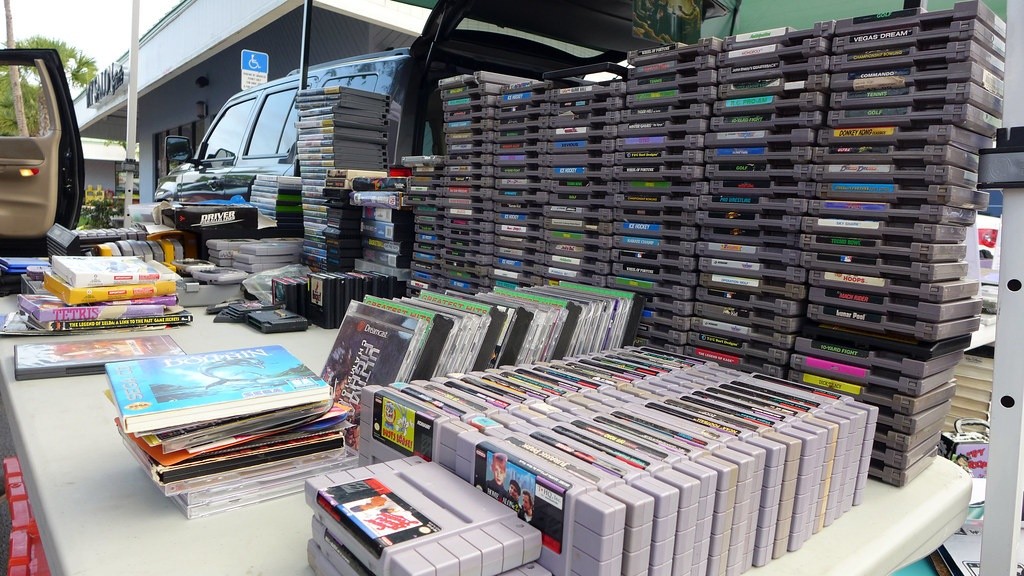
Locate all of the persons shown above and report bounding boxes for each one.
[484,453,533,525]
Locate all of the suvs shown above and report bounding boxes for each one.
[151,1,663,226]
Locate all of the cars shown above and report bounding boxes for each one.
[0,48,86,257]
